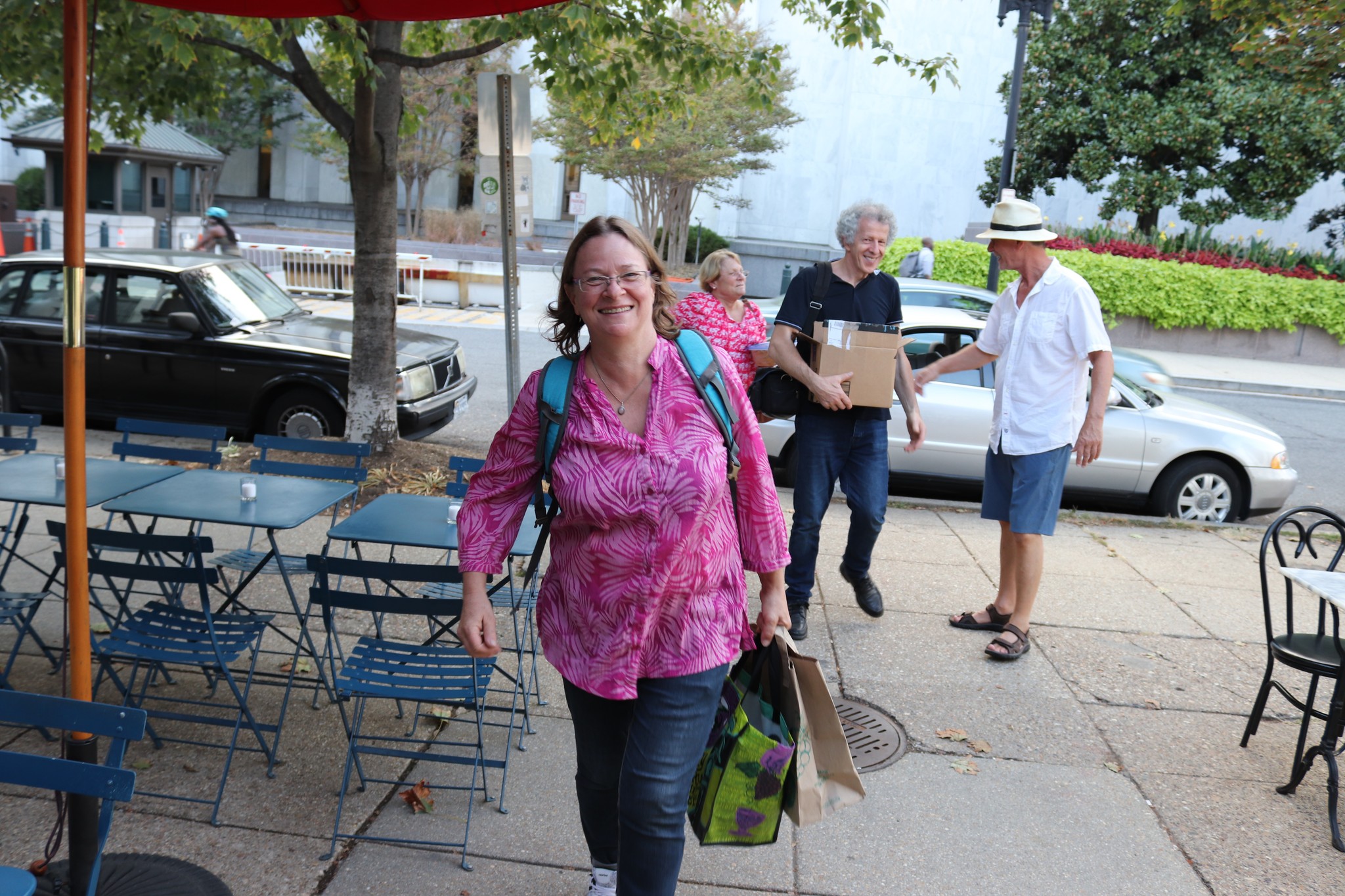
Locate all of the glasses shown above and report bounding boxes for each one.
[720,270,750,279]
[572,271,650,293]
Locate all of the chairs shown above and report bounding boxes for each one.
[929,340,953,358]
[1239,506,1345,798]
[0,411,569,896]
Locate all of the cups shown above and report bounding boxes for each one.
[239,476,257,501]
[447,498,465,524]
[54,456,65,480]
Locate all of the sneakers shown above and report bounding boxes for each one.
[588,866,616,896]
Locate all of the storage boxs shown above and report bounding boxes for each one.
[792,320,916,409]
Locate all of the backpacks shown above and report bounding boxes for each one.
[899,249,924,278]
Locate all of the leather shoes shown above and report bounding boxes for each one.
[839,561,884,618]
[787,605,810,639]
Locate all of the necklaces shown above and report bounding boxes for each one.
[587,343,653,415]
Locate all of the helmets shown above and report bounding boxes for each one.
[203,206,228,219]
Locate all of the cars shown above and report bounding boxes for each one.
[0,249,478,442]
[760,309,1300,525]
[749,275,1172,396]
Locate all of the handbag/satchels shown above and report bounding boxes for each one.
[685,623,866,848]
[748,367,808,420]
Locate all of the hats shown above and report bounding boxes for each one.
[976,198,1058,242]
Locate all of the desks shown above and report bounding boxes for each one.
[0,451,190,742]
[1276,566,1345,854]
[99,466,356,779]
[326,494,561,754]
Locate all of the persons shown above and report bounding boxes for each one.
[910,198,1114,662]
[769,202,924,642]
[189,206,241,273]
[457,213,795,895]
[914,236,934,278]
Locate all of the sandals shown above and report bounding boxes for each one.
[985,623,1031,659]
[948,604,1012,632]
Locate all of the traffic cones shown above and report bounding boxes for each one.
[22,217,36,252]
[197,227,204,252]
[0,225,6,256]
[116,229,125,249]
